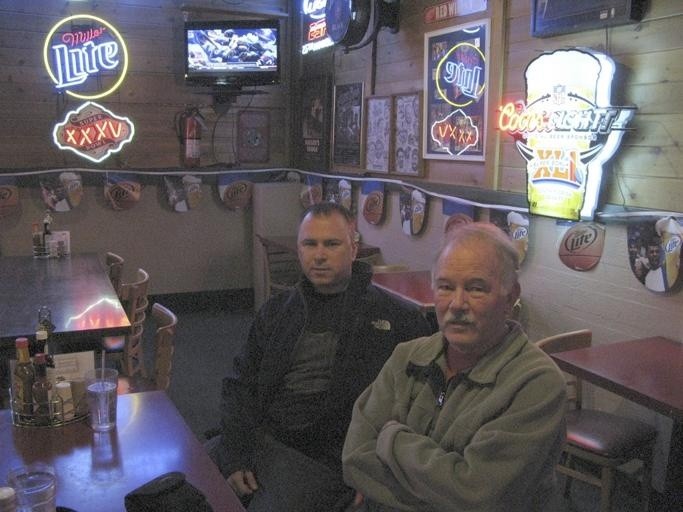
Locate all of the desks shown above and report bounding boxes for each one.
[278,236,380,261]
[549,336,682,512]
[1,252,132,406]
[1,390,244,512]
[372,270,438,336]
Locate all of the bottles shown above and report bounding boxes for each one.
[9,329,86,423]
[0,486,17,511]
[31,219,53,256]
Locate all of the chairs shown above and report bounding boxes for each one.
[71,268,149,377]
[537,329,658,510]
[260,234,303,304]
[150,301,177,396]
[105,252,124,294]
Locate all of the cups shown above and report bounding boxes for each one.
[516,49,608,220]
[64,176,82,206]
[84,365,120,434]
[339,185,353,212]
[182,180,201,209]
[412,197,426,235]
[662,230,681,291]
[7,461,57,512]
[511,221,529,262]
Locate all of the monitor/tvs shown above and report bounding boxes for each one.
[184,19,280,85]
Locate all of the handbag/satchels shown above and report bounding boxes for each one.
[125,470,216,512]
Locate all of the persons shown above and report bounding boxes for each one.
[340,222,567,510]
[626,223,680,291]
[202,203,433,511]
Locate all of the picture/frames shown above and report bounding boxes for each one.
[391,89,428,177]
[235,108,271,165]
[362,93,390,175]
[331,80,365,166]
[293,72,331,173]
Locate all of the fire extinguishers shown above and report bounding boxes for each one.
[175,106,207,169]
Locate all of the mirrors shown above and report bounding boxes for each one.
[422,14,488,164]
